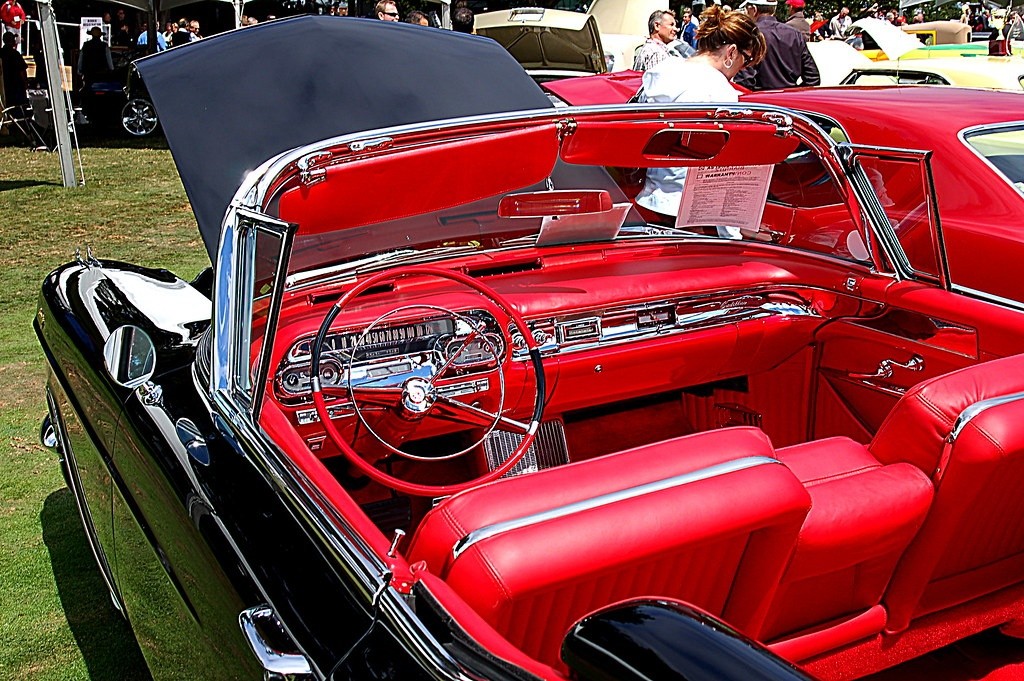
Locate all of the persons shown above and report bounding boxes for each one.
[632,4,768,240]
[0,1,475,143]
[632,0,1024,97]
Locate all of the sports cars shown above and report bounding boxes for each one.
[34,0,1024,681]
